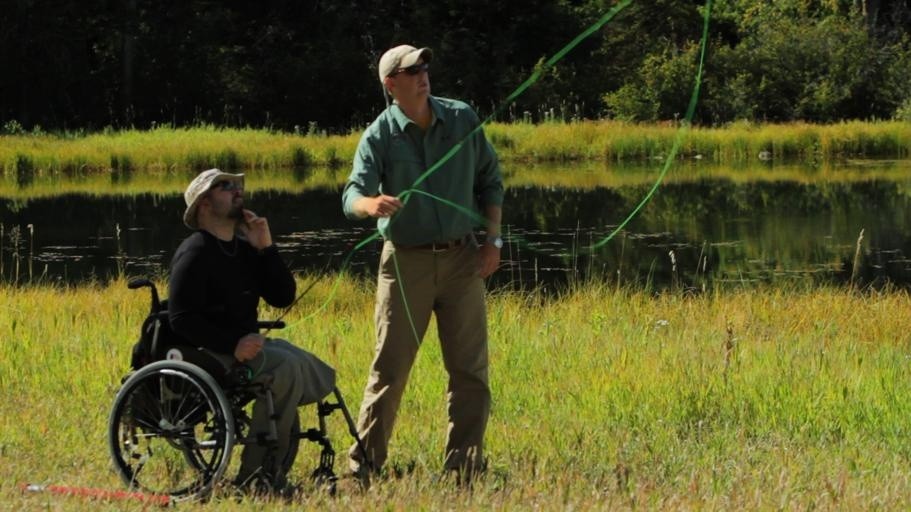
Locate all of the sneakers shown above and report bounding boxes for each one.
[440,467,479,489]
[231,476,301,503]
[344,470,370,494]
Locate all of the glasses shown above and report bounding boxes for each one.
[389,62,428,78]
[220,181,240,191]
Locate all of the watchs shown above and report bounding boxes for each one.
[482,234,504,249]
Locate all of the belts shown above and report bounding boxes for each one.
[418,236,472,252]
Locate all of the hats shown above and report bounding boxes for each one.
[379,45,433,83]
[184,168,245,230]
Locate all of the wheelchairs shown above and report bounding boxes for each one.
[108,276,375,501]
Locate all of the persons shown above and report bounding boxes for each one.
[165,168,337,502]
[340,45,506,490]
